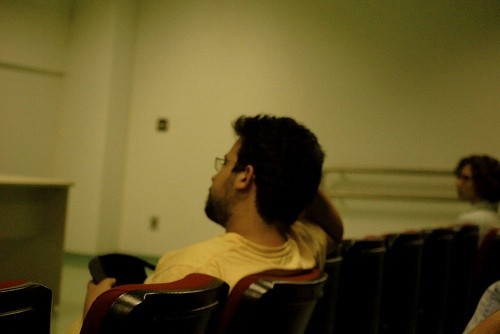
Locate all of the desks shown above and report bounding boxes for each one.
[0,171,74,309]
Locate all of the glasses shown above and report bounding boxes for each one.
[457,174,472,185]
[214,156,237,172]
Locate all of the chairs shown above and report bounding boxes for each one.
[1,217,499,334]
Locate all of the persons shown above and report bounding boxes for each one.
[81,111,331,325]
[451,153,500,248]
[463,279,500,334]
[296,183,346,257]
